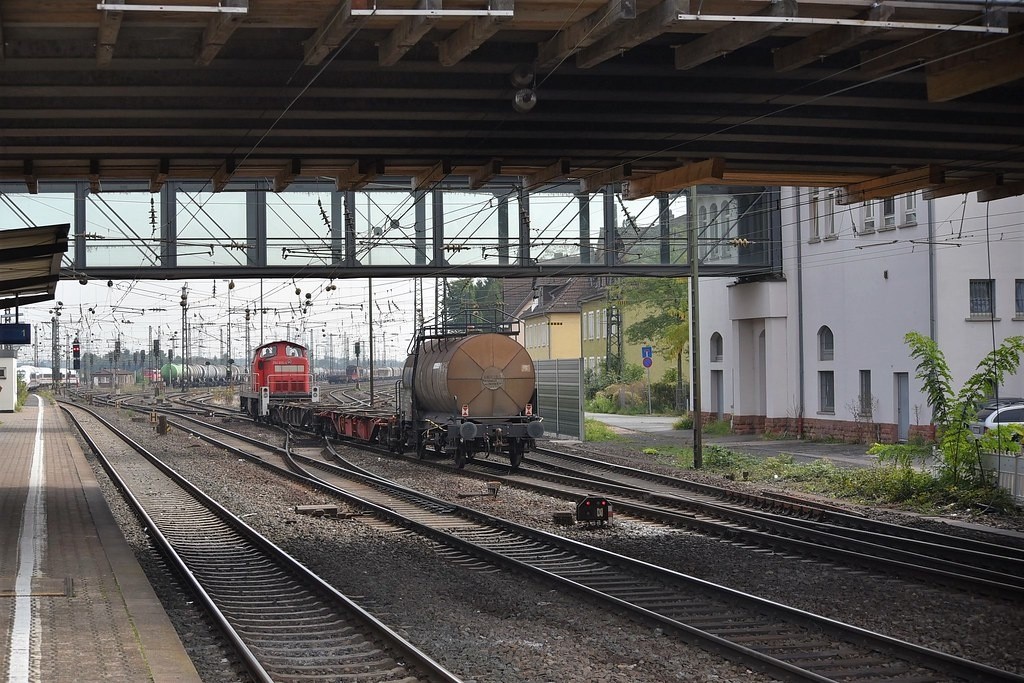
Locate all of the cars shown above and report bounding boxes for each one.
[968,404,1024,454]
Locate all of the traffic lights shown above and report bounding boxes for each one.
[73,345,80,358]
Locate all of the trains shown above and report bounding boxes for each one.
[161,362,402,389]
[237,308,546,468]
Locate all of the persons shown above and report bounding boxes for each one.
[264,349,272,355]
